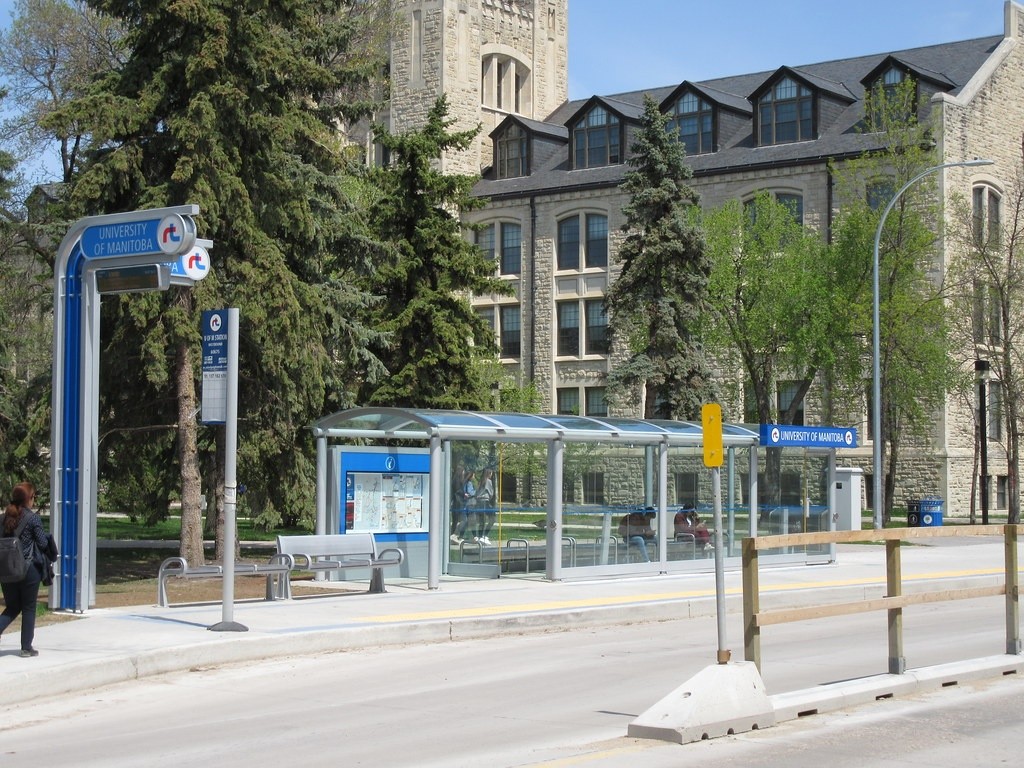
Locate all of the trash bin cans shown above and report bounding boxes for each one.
[904,499,945,527]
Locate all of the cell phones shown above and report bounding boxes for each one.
[703,520,706,525]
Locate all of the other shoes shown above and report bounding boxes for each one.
[704,544,715,551]
[450,534,461,544]
[474,536,478,542]
[21,647,38,657]
[479,536,492,545]
[458,538,469,545]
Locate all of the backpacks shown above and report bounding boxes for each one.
[617,513,641,536]
[0,511,35,584]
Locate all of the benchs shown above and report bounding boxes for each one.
[276,532,404,597]
[157,552,294,611]
[458,530,731,578]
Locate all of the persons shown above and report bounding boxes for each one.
[673,500,715,553]
[0,482,58,658]
[476,467,497,545]
[450,465,469,541]
[458,469,479,541]
[618,507,659,563]
